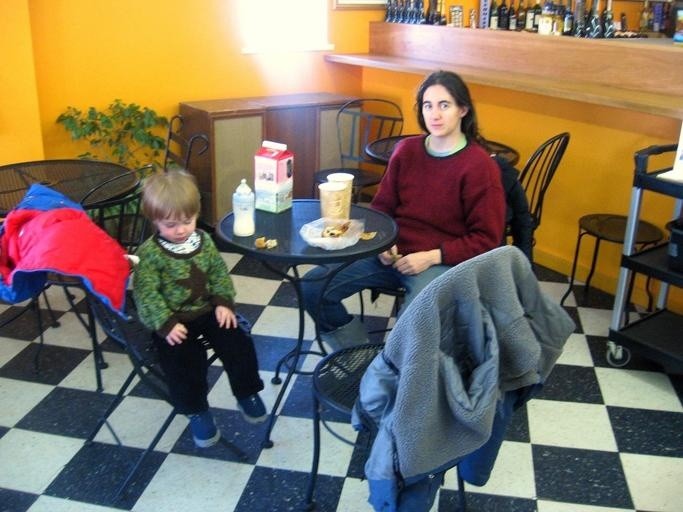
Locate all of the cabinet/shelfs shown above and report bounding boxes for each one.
[179,92,361,232]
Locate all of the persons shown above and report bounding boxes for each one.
[294,71,507,392]
[132,168,271,449]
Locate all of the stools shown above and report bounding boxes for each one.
[560,213,664,326]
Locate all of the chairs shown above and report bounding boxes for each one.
[79,278,251,504]
[301,245,574,512]
[376,132,571,294]
[310,98,404,204]
[2,115,211,394]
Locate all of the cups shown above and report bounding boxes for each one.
[319,172,355,228]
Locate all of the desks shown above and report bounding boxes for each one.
[364,135,520,171]
[218,198,400,450]
[1,158,141,368]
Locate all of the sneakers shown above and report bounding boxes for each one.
[189,408,221,450]
[235,391,268,424]
[315,349,378,400]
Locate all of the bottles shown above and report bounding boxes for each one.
[485,0,682,43]
[230,179,257,238]
[382,0,481,33]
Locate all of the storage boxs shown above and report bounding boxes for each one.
[666,220,682,274]
